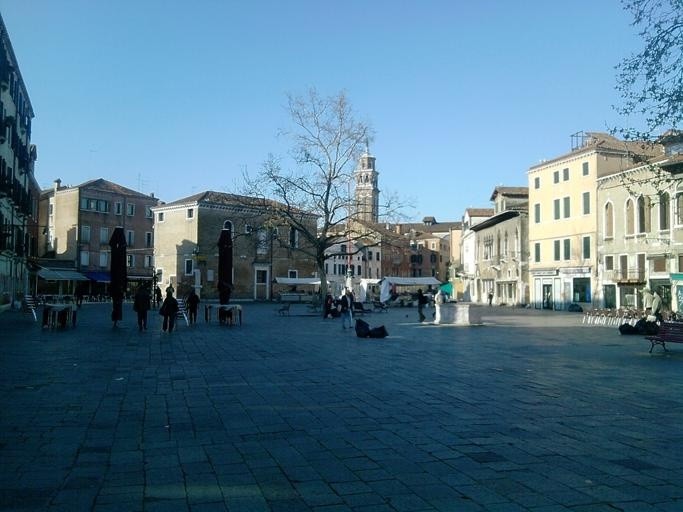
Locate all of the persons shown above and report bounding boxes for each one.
[165,283,174,297]
[433,288,442,322]
[323,290,356,322]
[486,288,494,305]
[156,287,161,308]
[132,283,153,331]
[158,292,178,332]
[417,288,426,322]
[340,289,353,329]
[185,287,200,325]
[651,290,663,316]
[73,281,84,309]
[638,287,653,314]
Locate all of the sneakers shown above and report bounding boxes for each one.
[139,327,148,330]
[418,316,425,322]
[343,326,353,329]
[190,321,196,325]
[161,327,172,331]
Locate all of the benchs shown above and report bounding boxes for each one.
[645,322,683,353]
[336,300,387,317]
